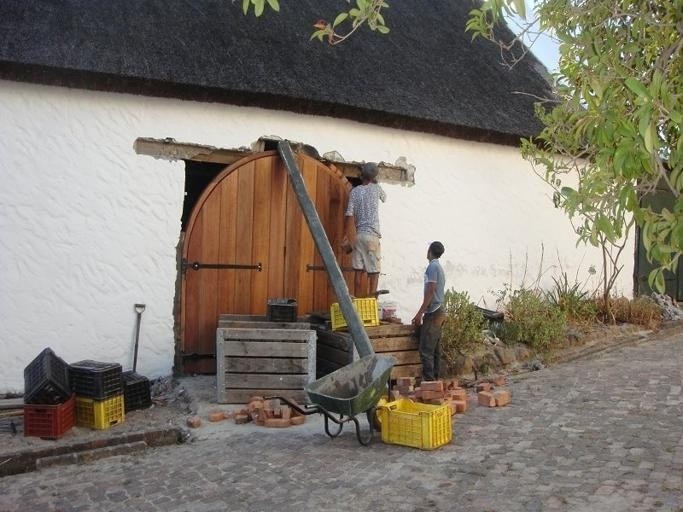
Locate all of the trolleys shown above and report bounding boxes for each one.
[262,353,396,446]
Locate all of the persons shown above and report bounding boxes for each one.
[412,241,445,381]
[342,162,387,299]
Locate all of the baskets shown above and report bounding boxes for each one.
[331,298,380,332]
[376,398,453,451]
[267,298,297,322]
[24,346,153,439]
